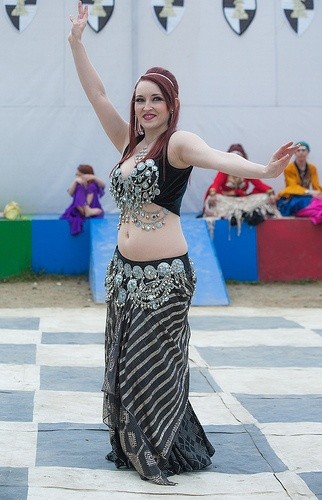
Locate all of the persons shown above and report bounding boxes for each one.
[68,164,106,217]
[69,0,301,486]
[277,141,322,216]
[203,143,276,215]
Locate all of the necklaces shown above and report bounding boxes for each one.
[134,135,161,165]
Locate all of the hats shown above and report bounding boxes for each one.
[293,140,310,151]
